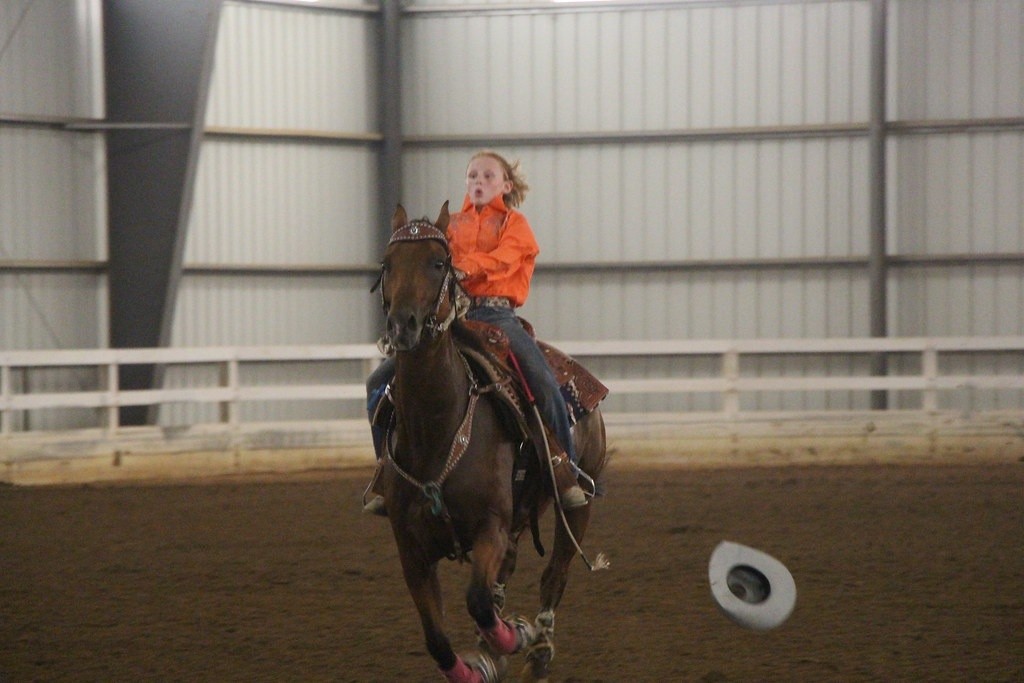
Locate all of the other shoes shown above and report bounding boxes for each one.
[561,482,589,511]
[362,494,386,518]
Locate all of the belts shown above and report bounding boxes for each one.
[467,295,514,308]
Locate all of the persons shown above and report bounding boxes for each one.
[351,145,591,517]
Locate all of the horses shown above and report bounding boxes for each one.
[377,198,619,683]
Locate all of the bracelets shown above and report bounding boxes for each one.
[459,269,467,280]
